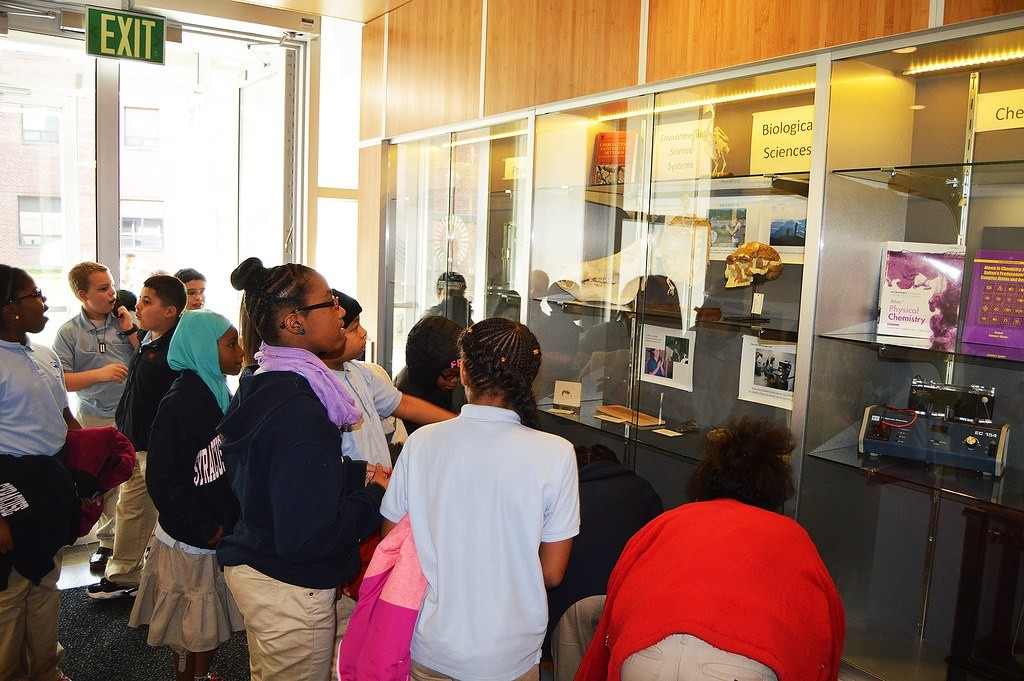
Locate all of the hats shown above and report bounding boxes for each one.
[332,289,362,330]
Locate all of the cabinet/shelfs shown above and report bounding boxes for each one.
[389,156,1024,472]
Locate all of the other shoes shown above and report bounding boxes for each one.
[195,670,226,681]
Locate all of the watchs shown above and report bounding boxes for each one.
[122,323,138,336]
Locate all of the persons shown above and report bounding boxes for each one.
[52,261,155,569]
[521,269,743,430]
[86,275,188,599]
[726,218,741,248]
[429,272,474,328]
[548,444,667,681]
[175,268,207,310]
[665,339,679,361]
[386,316,468,468]
[0,263,106,681]
[647,348,665,377]
[756,353,774,378]
[320,288,458,681]
[379,318,580,681]
[214,257,391,681]
[128,308,245,643]
[573,419,846,681]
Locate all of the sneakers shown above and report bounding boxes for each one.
[89,547,113,567]
[86,578,139,599]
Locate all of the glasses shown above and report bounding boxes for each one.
[280,298,338,328]
[2,288,42,312]
[441,371,460,380]
